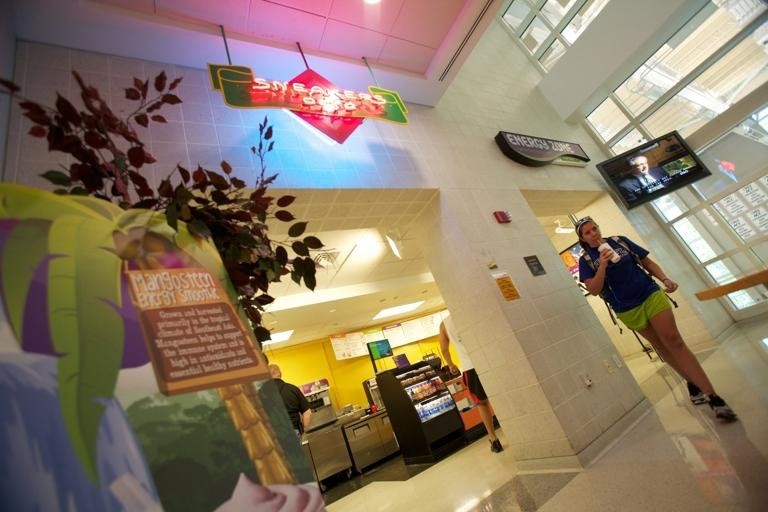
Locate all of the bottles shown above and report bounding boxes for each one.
[404,377,456,424]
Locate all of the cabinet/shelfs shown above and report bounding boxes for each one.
[375,360,467,465]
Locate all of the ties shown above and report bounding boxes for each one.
[642,175,657,187]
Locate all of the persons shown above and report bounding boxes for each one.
[258,363,313,448]
[618,153,673,204]
[439,313,505,453]
[213,471,326,512]
[675,160,690,172]
[574,213,740,424]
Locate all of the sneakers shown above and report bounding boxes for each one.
[687,383,734,418]
[489,439,502,452]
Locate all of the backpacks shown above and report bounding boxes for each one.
[582,235,641,302]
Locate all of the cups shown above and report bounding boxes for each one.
[365,406,379,415]
[597,241,621,264]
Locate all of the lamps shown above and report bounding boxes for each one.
[385,225,408,260]
[554,219,575,234]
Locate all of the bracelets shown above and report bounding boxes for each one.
[662,278,669,283]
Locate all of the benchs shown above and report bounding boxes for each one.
[695,267,768,301]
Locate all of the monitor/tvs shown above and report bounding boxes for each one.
[368,338,393,361]
[596,129,712,210]
